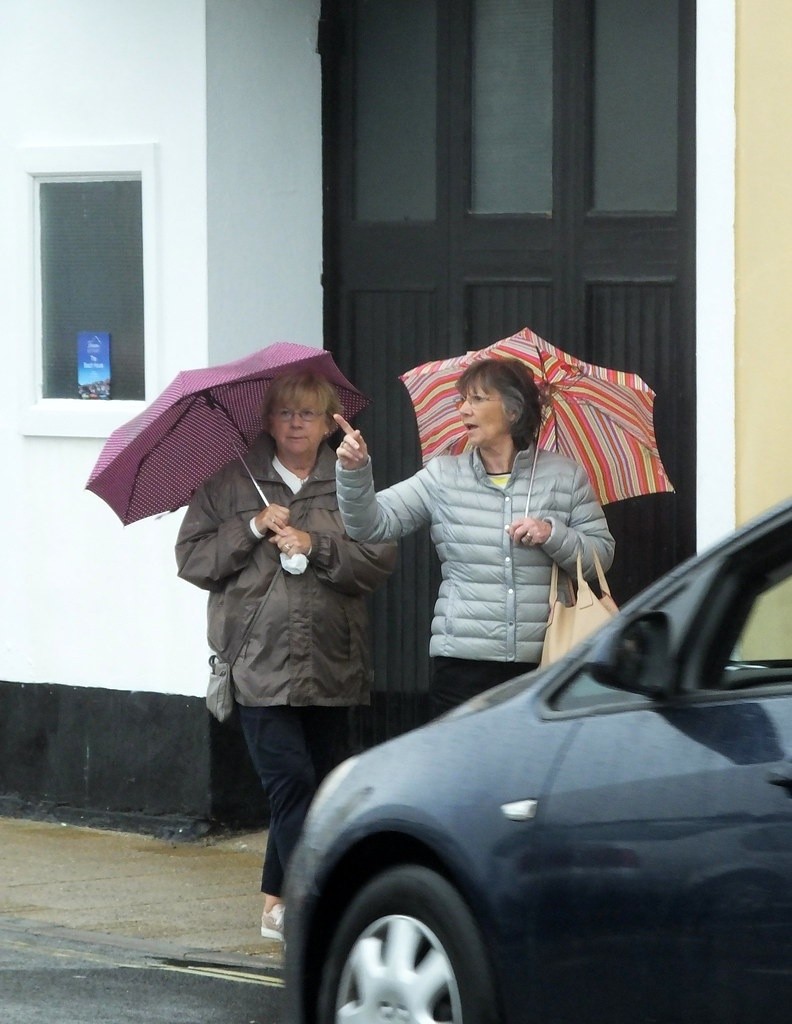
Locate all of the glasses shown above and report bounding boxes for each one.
[269,407,324,422]
[453,395,501,410]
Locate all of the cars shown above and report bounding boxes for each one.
[284,500,792,1023]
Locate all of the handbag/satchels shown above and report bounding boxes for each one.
[540,542,619,668]
[206,655,233,723]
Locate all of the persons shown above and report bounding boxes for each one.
[177,371,395,937]
[332,360,615,713]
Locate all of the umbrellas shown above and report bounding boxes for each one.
[399,328,675,516]
[84,341,373,526]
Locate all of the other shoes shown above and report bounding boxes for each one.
[261,904,285,941]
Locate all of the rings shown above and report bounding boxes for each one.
[285,545,290,549]
[527,533,532,537]
